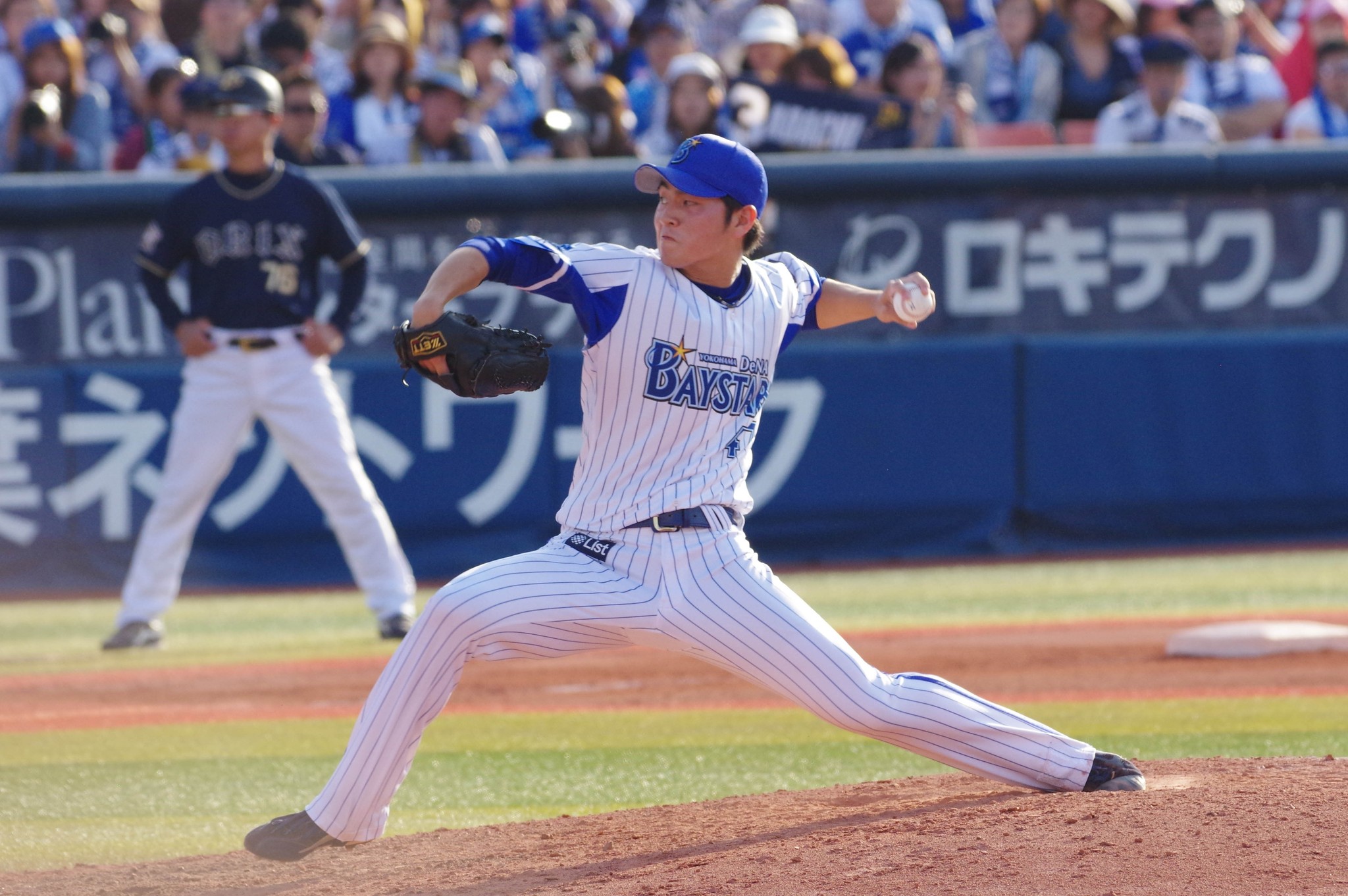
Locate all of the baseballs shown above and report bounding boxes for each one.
[892,282,934,324]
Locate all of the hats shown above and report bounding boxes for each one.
[20,21,69,47]
[1137,37,1189,63]
[739,6,796,48]
[358,14,408,49]
[460,13,502,56]
[203,65,285,116]
[418,56,475,98]
[663,54,716,86]
[634,134,768,220]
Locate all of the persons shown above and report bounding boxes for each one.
[243,136,1147,861]
[102,66,418,649]
[0,0,1348,174]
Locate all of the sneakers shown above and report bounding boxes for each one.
[244,811,372,861]
[381,612,413,638]
[1085,752,1142,794]
[101,623,159,647]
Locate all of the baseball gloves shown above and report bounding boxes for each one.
[394,311,549,400]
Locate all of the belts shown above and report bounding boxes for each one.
[621,506,736,532]
[204,334,301,351]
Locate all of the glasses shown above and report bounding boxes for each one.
[287,100,326,116]
